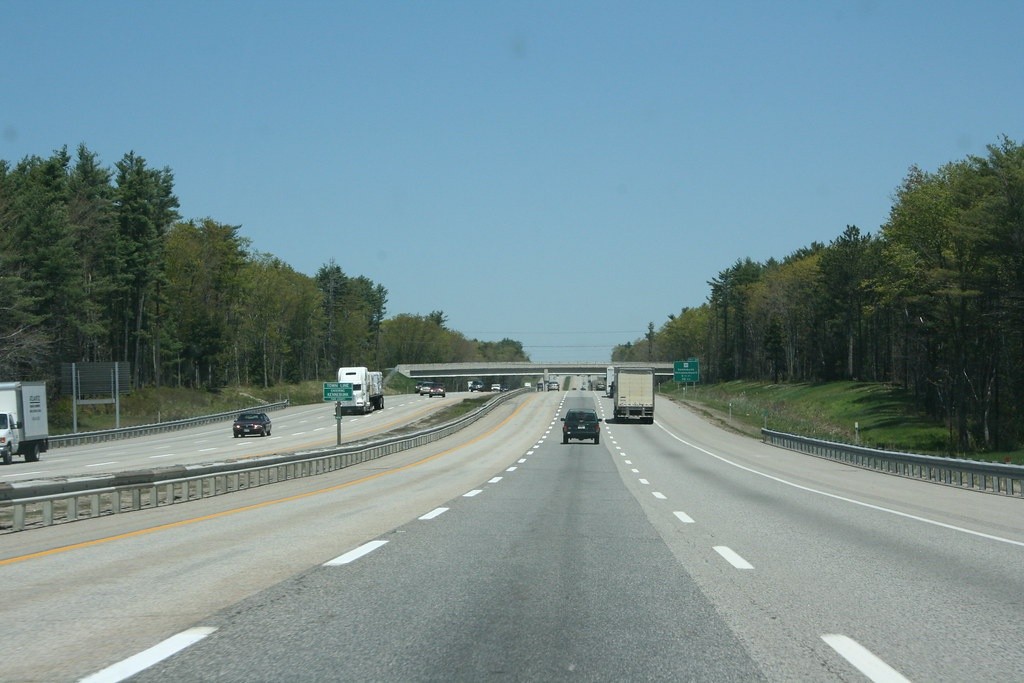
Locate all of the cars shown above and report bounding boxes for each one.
[524,382,532,390]
[570,384,606,391]
[233,412,272,437]
[414,381,424,394]
[490,383,501,393]
[500,384,513,392]
[469,381,485,393]
[537,382,543,388]
[547,382,560,391]
[609,381,613,398]
[560,409,603,445]
[418,380,434,397]
[428,384,446,397]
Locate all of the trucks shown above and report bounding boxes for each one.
[609,365,655,425]
[0,381,50,464]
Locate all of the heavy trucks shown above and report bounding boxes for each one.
[333,367,385,415]
